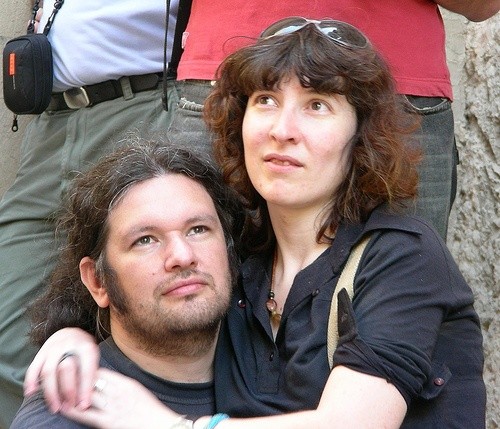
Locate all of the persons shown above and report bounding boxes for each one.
[160,1,499,250]
[10,141,255,429]
[0,1,193,399]
[24,17,487,429]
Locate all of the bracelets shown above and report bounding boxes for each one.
[202,413,230,429]
[177,412,200,428]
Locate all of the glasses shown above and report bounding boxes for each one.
[258,15,369,49]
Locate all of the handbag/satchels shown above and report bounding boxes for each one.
[3,33,53,132]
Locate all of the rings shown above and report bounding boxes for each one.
[54,352,77,364]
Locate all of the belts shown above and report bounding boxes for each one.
[45,71,176,110]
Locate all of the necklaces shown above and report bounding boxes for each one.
[266,247,282,325]
